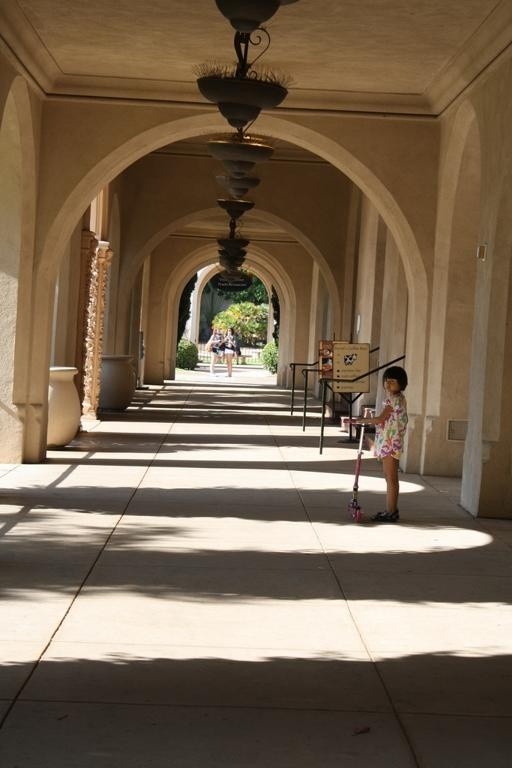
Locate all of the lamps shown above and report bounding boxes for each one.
[186,1,290,277]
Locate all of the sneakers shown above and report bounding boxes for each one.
[371,510,399,522]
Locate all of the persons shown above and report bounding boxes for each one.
[348,365,408,524]
[208,327,224,376]
[222,328,237,378]
